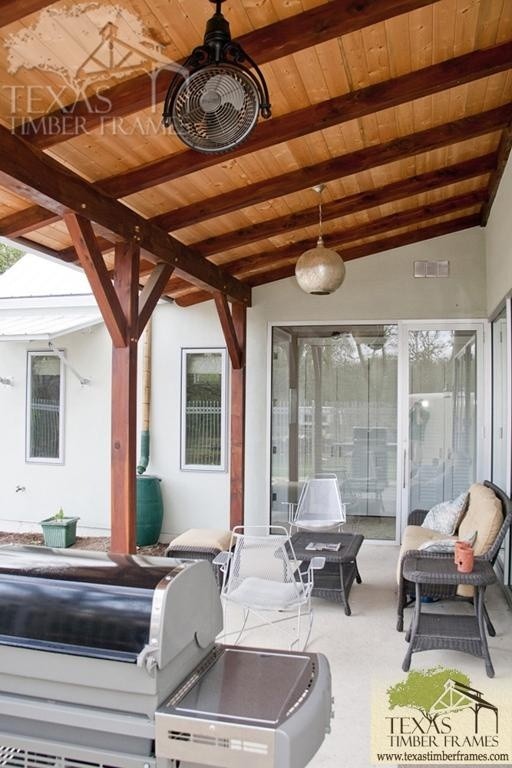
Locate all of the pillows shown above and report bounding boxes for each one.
[418,530,478,553]
[420,491,469,534]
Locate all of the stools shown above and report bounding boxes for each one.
[163,526,239,589]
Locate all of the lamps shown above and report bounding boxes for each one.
[293,185,346,294]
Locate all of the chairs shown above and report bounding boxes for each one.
[278,427,398,522]
[211,523,329,656]
[281,469,349,537]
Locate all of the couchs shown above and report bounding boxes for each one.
[412,451,473,513]
[395,478,512,634]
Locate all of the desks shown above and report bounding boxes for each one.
[402,553,499,678]
[276,527,364,618]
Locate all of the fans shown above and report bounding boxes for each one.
[161,0,273,153]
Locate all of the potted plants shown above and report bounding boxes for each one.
[39,508,80,548]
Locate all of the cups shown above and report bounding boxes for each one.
[457,548,473,575]
[456,541,470,565]
